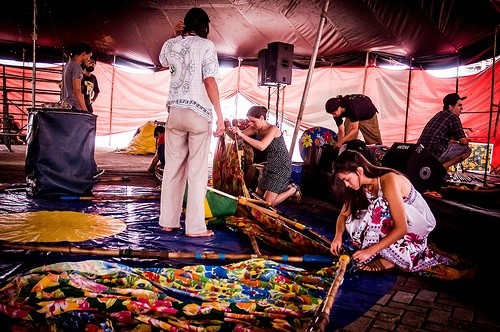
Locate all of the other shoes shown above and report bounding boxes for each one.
[93,169,105,179]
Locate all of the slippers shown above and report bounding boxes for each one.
[364,260,396,272]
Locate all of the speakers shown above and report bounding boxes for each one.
[381,142,448,193]
[256,41,294,87]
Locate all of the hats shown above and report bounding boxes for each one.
[443,93,467,105]
[325,95,343,114]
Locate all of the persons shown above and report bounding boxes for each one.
[9,116,20,128]
[62,42,105,180]
[418,94,472,169]
[148,126,165,171]
[325,92,383,149]
[330,151,451,272]
[224,105,303,207]
[159,7,224,237]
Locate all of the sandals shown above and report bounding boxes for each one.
[290,183,302,205]
